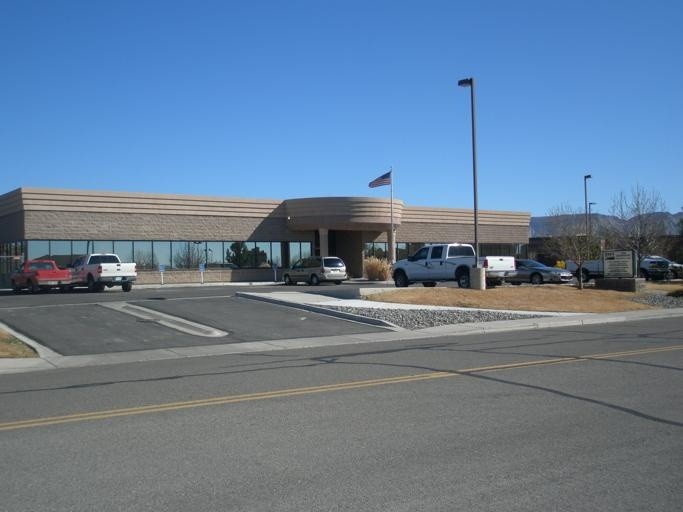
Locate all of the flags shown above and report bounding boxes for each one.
[368,171,392,187]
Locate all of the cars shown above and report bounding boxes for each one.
[647,258,682,280]
[206,263,239,269]
[281,256,347,286]
[493,258,573,286]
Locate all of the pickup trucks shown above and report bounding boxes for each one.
[69,252,137,293]
[390,243,517,288]
[10,260,71,294]
[564,253,668,284]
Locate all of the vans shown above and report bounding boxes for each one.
[534,254,579,277]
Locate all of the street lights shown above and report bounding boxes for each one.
[193,240,201,269]
[588,202,596,258]
[457,77,479,267]
[583,174,592,258]
